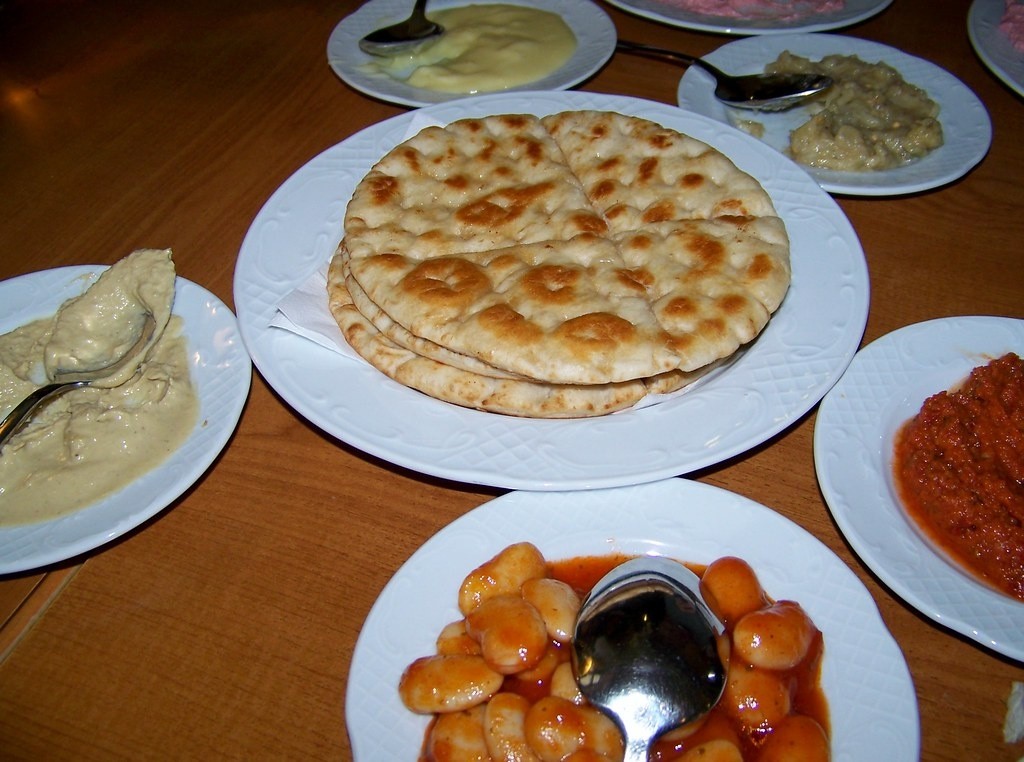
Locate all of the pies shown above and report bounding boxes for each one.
[326,107,795,420]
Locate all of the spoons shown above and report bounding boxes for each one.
[613,39,834,111]
[358,0,446,58]
[0,315,159,452]
[568,556,733,762]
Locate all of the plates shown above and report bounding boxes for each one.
[233,90,868,492]
[342,475,921,762]
[0,264,254,574]
[675,33,993,196]
[605,0,894,37]
[967,0,1024,100]
[326,0,618,108]
[813,316,1024,665]
